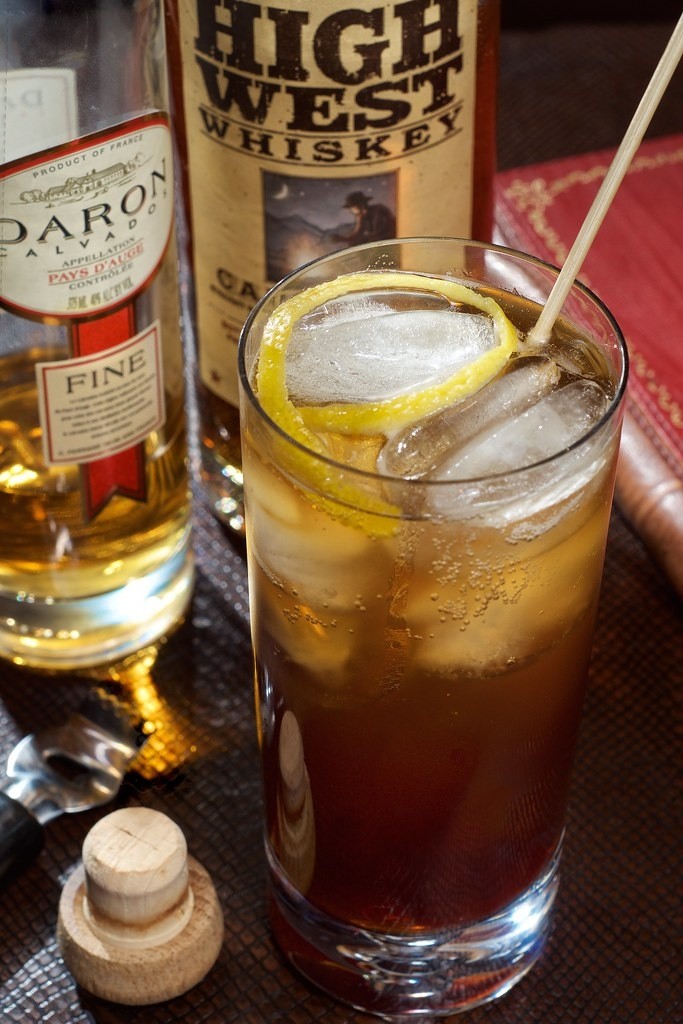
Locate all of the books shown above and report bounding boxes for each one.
[491,132,682,576]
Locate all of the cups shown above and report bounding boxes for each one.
[235,239,629,1024]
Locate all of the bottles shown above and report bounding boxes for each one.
[167,0,500,534]
[0,0,188,670]
